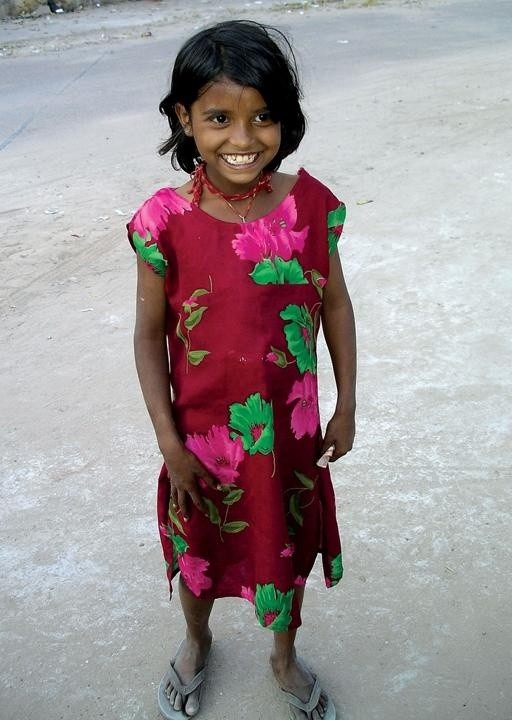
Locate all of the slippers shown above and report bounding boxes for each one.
[158,639,208,720]
[280,655,336,720]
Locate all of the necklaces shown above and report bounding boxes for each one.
[185,160,276,224]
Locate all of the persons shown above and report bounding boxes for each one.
[123,19,360,720]
